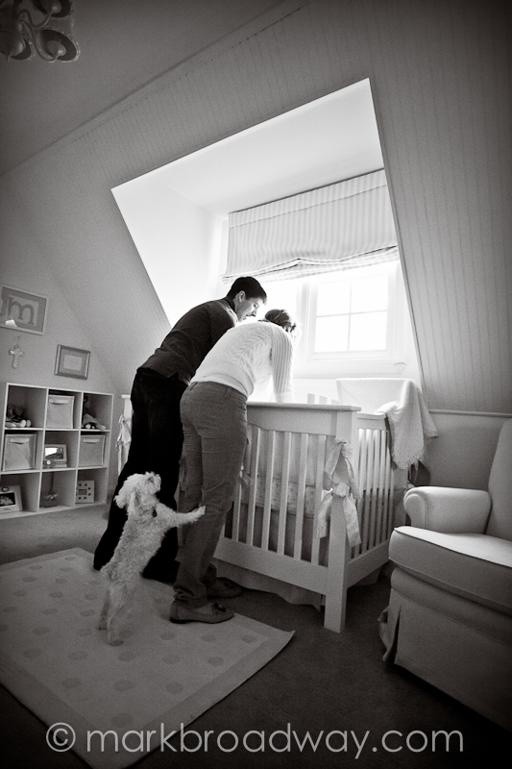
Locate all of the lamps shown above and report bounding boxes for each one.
[0,0,80,63]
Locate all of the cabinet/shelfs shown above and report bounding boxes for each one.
[0,383,114,523]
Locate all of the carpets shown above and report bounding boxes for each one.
[1,548,296,769]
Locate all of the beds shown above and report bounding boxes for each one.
[117,393,426,634]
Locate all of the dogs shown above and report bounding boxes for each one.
[96,471,207,647]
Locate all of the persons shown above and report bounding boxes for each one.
[170,310,297,624]
[94,277,266,584]
[9,302,33,326]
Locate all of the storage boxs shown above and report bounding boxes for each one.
[46,395,75,430]
[75,480,96,503]
[80,435,105,467]
[2,433,37,471]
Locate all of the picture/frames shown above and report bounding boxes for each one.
[0,284,50,336]
[54,344,91,381]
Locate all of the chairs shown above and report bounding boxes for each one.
[376,418,511,734]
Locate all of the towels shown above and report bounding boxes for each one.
[374,380,438,470]
